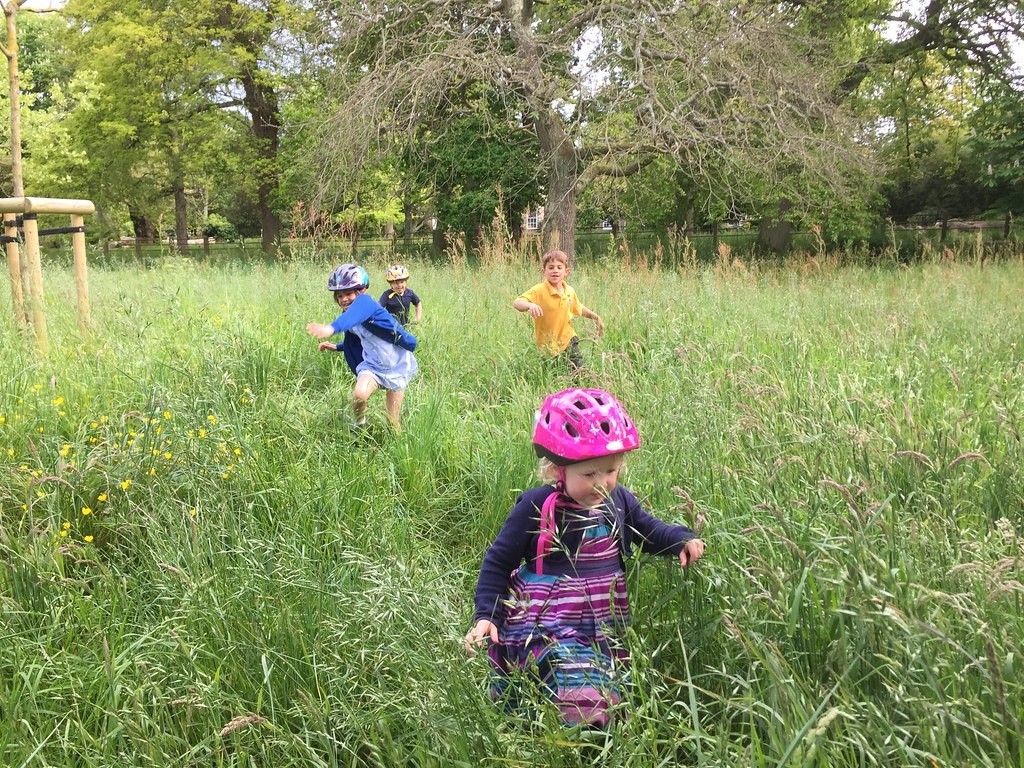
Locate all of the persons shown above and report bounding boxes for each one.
[305,263,418,447]
[377,265,422,331]
[463,386,705,768]
[513,251,605,388]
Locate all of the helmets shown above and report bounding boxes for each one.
[328,264,370,292]
[532,389,640,467]
[386,264,409,282]
